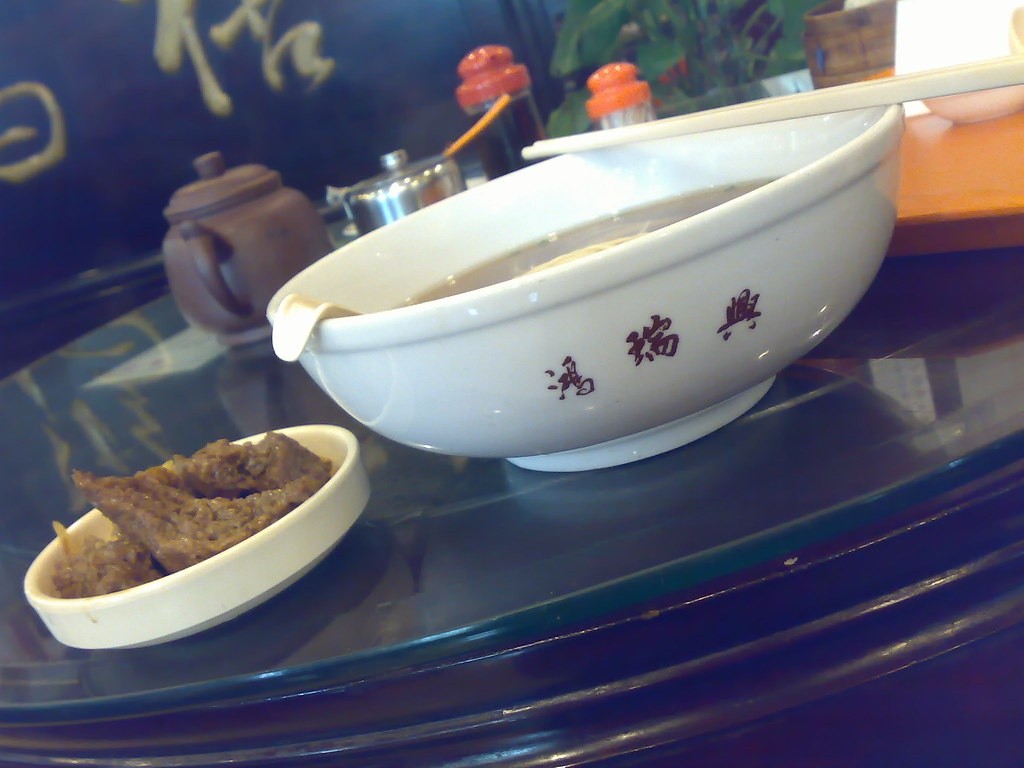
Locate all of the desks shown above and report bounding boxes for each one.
[0,194,1024,768]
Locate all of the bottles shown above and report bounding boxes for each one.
[455,45,550,179]
[585,61,657,130]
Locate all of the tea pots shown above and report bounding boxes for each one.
[162,150,336,347]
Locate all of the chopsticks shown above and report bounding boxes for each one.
[521,50,1023,160]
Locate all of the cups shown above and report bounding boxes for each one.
[340,148,468,236]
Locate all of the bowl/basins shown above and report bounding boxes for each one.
[23,424,372,650]
[264,102,905,473]
